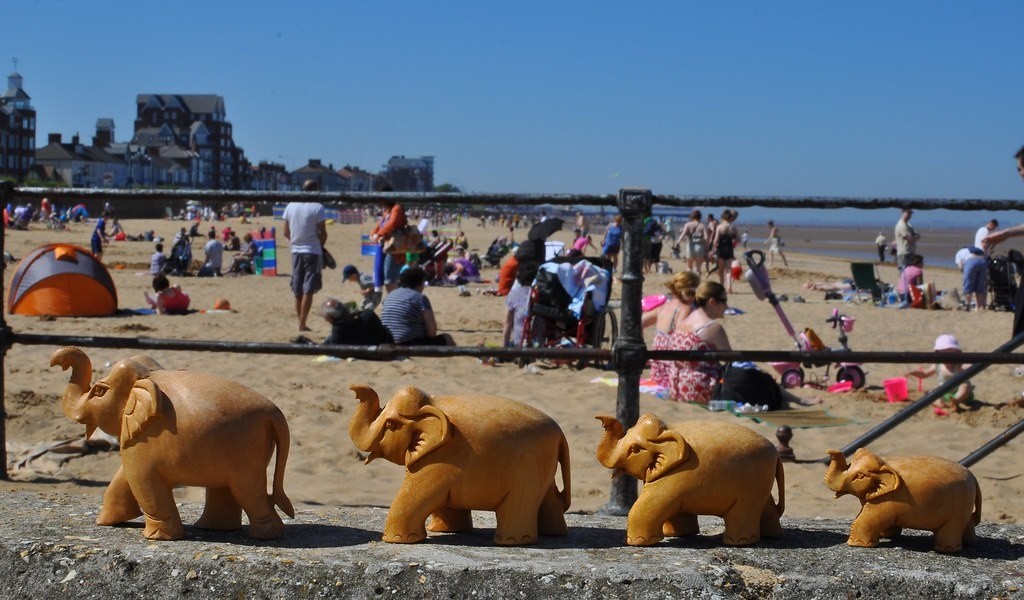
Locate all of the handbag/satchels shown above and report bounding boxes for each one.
[936,287,964,312]
[382,203,425,255]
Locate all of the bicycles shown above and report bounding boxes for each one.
[743,249,869,392]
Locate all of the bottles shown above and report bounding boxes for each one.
[708,400,743,412]
[657,388,671,400]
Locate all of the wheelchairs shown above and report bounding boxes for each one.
[518,255,619,368]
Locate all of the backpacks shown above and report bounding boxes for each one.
[711,362,790,411]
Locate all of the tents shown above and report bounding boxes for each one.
[7,243,118,316]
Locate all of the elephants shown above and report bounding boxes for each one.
[350,384,571,546]
[49,346,294,539]
[826,449,983,550]
[597,414,785,547]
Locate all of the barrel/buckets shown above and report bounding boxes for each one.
[883,378,908,402]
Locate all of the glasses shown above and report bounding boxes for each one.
[719,298,728,305]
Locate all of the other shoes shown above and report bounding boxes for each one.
[321,247,336,270]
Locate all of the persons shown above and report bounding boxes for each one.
[0,146,1024,412]
[151,275,190,315]
[282,179,327,333]
[902,334,975,413]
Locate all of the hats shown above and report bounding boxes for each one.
[902,206,914,212]
[341,265,357,283]
[934,334,961,351]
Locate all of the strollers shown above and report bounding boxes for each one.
[987,255,1019,312]
